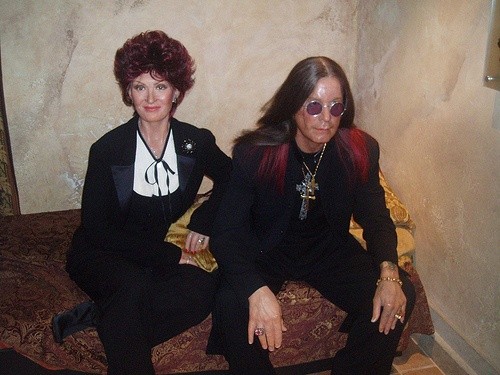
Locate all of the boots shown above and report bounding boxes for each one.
[51,303,96,343]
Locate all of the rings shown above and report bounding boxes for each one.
[198,238,204,243]
[254,327,265,336]
[395,312,403,320]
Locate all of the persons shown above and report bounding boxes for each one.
[50,29,233,375]
[208,56,418,375]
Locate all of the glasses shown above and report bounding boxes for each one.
[302,100,346,117]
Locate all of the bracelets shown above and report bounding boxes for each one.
[376,276,403,287]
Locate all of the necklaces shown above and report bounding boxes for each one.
[290,142,327,221]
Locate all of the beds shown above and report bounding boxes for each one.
[0,194,436,375]
